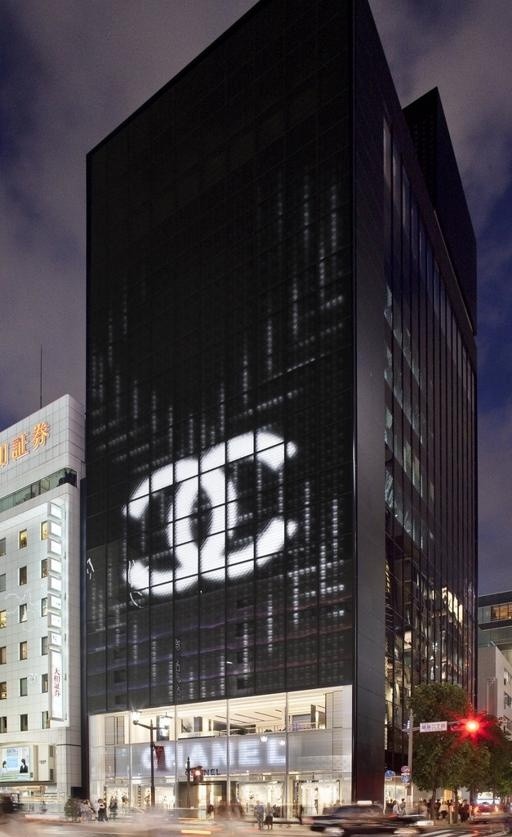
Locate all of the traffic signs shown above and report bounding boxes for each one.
[418,722,448,733]
[401,773,411,784]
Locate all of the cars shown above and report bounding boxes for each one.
[309,802,434,836]
[0,792,21,818]
[468,809,512,825]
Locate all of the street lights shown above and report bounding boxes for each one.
[184,756,204,809]
[132,719,157,811]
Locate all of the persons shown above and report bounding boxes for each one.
[254,799,265,830]
[79,798,119,824]
[385,797,480,824]
[265,802,274,830]
[206,803,214,818]
[40,800,47,814]
[1,759,8,772]
[298,803,304,824]
[20,758,29,773]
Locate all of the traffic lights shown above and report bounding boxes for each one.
[451,719,481,733]
